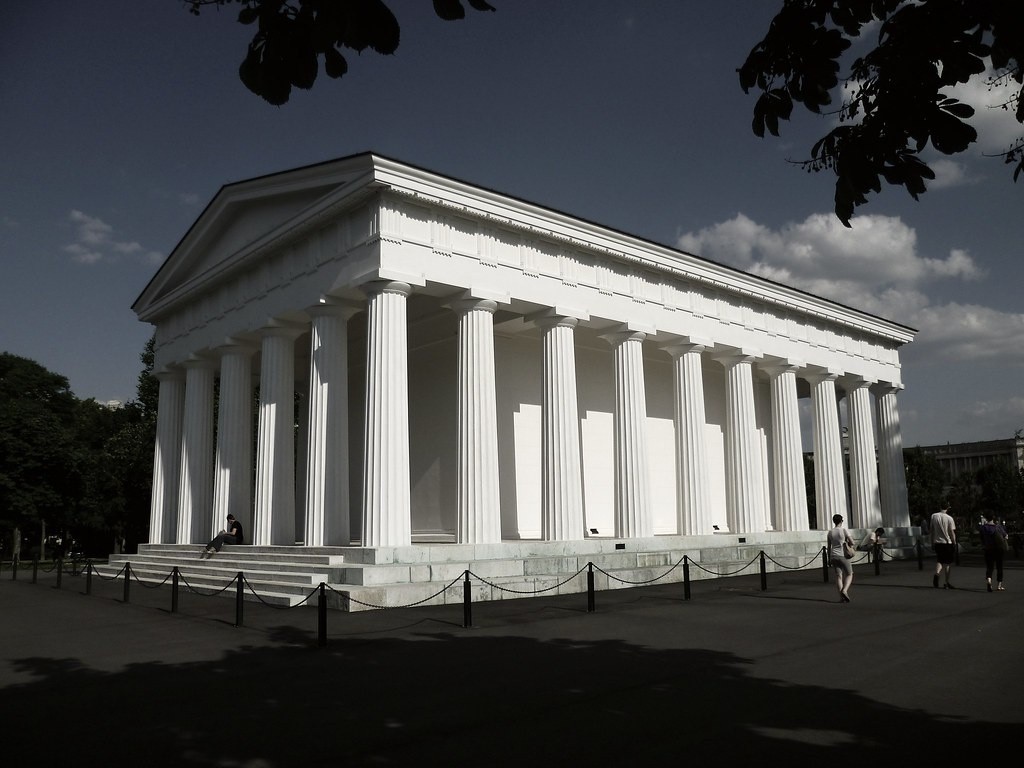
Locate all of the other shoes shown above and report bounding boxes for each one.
[200,547,216,553]
[944,582,956,589]
[933,573,939,587]
[840,591,850,603]
[987,583,992,592]
[997,586,1005,591]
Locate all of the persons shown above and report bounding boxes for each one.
[993,518,1019,533]
[858,527,887,562]
[200,514,243,554]
[827,515,854,603]
[920,516,929,536]
[930,501,959,590]
[980,510,1006,592]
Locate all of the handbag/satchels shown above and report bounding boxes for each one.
[843,542,856,559]
[995,530,1009,551]
